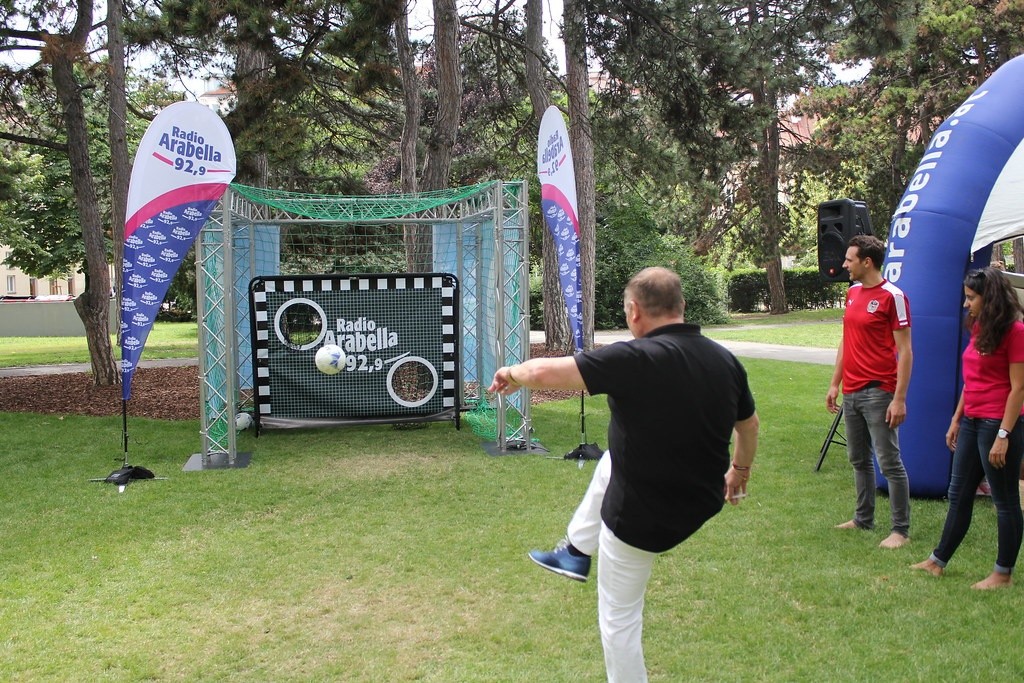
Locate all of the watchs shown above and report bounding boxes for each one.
[998,428,1011,439]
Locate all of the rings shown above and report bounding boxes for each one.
[505,386,508,390]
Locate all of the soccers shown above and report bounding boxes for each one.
[315,345,346,375]
[235,413,253,431]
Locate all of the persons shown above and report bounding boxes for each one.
[826,234,914,549]
[990,261,1005,271]
[909,266,1024,589]
[488,264,759,683]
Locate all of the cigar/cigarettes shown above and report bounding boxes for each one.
[725,494,746,499]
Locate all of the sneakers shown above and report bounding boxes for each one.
[528,536,591,583]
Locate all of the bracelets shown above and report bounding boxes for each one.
[732,460,751,480]
[506,364,522,388]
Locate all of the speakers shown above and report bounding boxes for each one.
[818,198,873,283]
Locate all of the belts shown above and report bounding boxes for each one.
[861,380,881,390]
